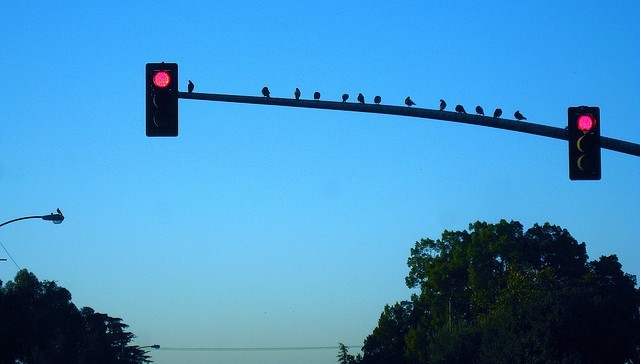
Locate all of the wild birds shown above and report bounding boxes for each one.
[455,104,467,113]
[494,108,503,117]
[475,105,484,115]
[342,93,349,102]
[357,92,364,102]
[261,86,271,96]
[404,95,417,107]
[514,109,527,121]
[374,95,381,105]
[439,98,447,110]
[187,79,195,92]
[293,87,301,99]
[313,91,321,99]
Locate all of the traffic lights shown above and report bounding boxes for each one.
[145,62,178,137]
[567,105,600,179]
